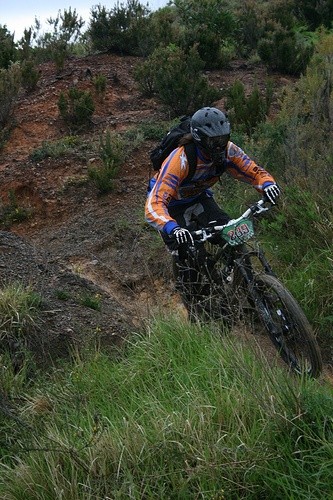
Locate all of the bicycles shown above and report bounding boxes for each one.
[171,198,323,381]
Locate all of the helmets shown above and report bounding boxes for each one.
[191,106,230,160]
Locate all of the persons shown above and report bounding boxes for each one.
[143,107,282,324]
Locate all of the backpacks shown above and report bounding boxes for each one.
[150,115,228,188]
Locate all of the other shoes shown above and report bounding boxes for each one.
[246,291,280,309]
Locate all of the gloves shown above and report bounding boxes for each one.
[263,184,281,205]
[170,226,194,247]
